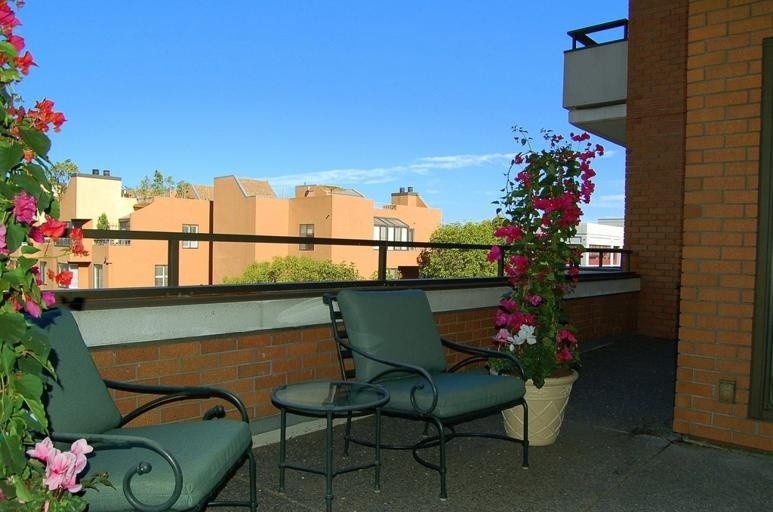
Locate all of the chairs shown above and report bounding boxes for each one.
[319,289,529,506]
[12,304,261,512]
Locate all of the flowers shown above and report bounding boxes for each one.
[16,290,57,322]
[482,121,603,388]
[21,430,93,508]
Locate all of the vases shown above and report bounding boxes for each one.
[491,370,578,448]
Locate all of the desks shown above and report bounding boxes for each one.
[268,380,393,509]
